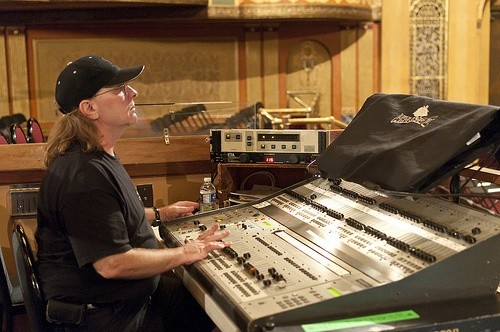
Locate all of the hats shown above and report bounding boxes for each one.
[55,56,145,115]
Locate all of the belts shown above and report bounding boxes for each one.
[84,302,125,309]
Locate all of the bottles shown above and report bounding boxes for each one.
[198,177,216,213]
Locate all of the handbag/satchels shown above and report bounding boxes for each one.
[316,93,500,194]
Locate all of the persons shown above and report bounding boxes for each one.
[34,55,231,332]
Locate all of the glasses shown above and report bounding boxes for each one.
[87,82,127,99]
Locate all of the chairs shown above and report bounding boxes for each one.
[0,118,44,144]
[12,223,47,331]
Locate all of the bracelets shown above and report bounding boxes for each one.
[153,207,160,227]
